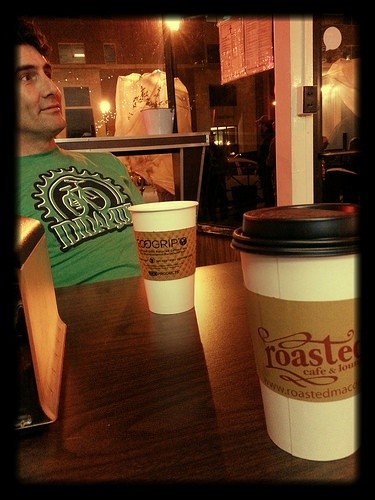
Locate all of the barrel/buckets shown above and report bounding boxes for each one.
[141,107,175,135]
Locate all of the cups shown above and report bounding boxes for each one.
[230,203,360,460]
[127,201,200,314]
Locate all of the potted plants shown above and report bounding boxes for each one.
[128,86,175,135]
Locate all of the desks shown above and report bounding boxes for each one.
[18,261,357,481]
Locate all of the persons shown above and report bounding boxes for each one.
[16,20,145,288]
